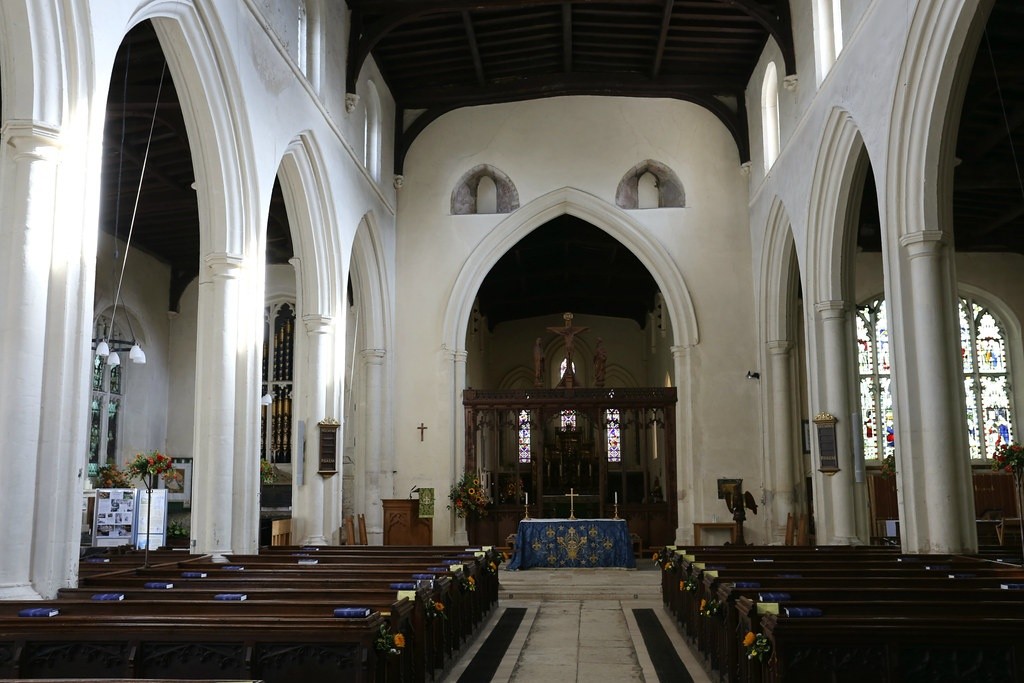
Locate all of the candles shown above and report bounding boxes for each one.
[614,492,618,504]
[525,492,529,505]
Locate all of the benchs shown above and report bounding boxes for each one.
[0,544,509,683]
[661,544,1024,683]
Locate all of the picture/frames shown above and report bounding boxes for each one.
[802,419,812,454]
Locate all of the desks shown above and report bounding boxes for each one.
[506,518,636,572]
[692,521,737,546]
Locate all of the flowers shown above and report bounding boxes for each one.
[506,478,524,498]
[128,451,174,482]
[447,472,491,520]
[95,465,131,487]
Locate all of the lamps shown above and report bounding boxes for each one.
[90,37,148,366]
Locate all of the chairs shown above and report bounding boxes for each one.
[345,514,369,546]
[784,512,810,546]
